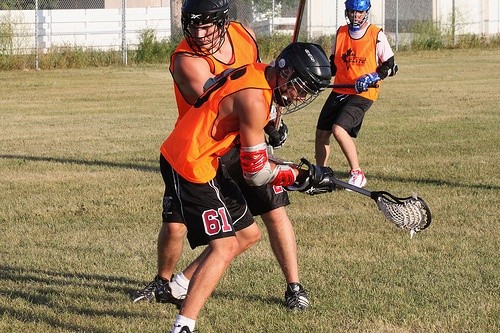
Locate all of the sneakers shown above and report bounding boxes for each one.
[155,283,189,309]
[170,326,192,333]
[345,169,367,191]
[282,282,309,310]
[132,274,174,304]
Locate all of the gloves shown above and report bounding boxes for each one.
[264,118,290,147]
[282,157,337,195]
[355,72,380,94]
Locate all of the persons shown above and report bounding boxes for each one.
[315,0,398,191]
[131,0,310,310]
[158,40,339,333]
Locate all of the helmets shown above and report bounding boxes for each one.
[276,42,331,106]
[181,0,229,28]
[345,0,371,31]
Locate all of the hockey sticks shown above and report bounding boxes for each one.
[275,0,306,132]
[267,156,432,233]
[319,84,380,88]
